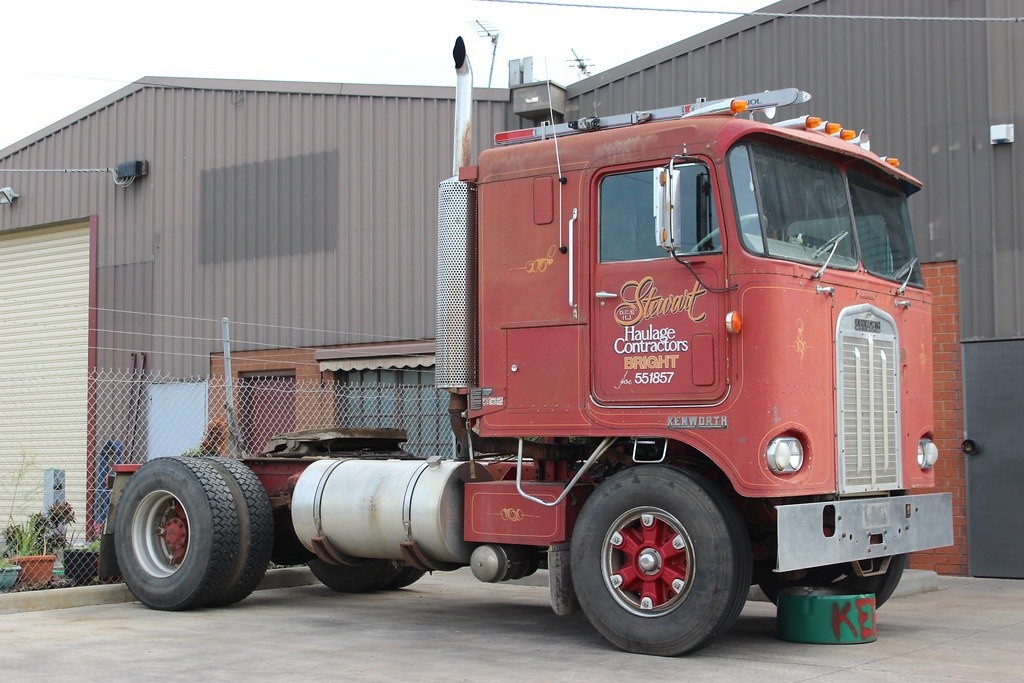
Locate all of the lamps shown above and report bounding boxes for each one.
[0,187,20,204]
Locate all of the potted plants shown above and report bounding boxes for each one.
[0,512,101,590]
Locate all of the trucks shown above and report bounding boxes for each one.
[88,33,957,659]
